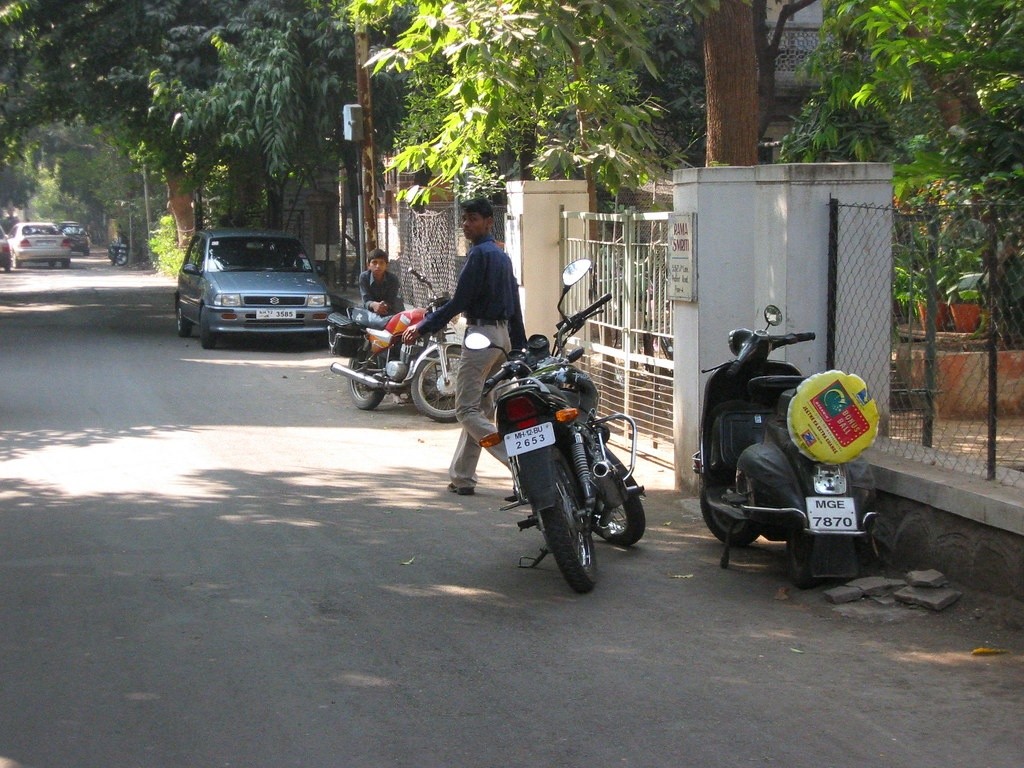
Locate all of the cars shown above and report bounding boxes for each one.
[55,219,91,257]
[0,224,13,274]
[6,223,71,270]
[175,228,330,351]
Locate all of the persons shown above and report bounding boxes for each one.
[402,198,527,495]
[359,248,412,405]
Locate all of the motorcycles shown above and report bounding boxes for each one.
[463,257,648,593]
[107,226,130,267]
[327,266,466,423]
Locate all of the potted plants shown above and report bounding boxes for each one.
[902,242,949,332]
[946,246,982,332]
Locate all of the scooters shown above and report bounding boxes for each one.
[690,304,880,592]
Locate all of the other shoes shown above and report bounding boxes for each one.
[447,482,476,495]
[505,491,520,502]
